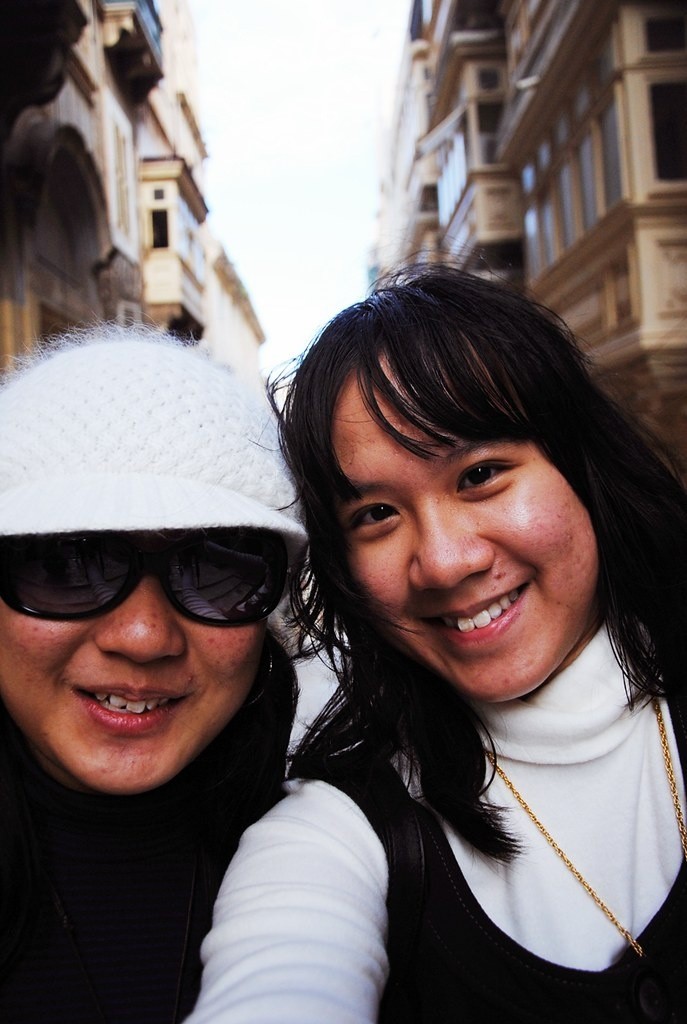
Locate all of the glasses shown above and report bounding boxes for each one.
[0,527,295,626]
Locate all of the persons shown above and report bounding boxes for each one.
[0,325,345,1023]
[185,267,687,1023]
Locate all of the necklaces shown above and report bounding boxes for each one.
[487,692,687,957]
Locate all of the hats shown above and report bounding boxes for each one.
[0,316,309,552]
